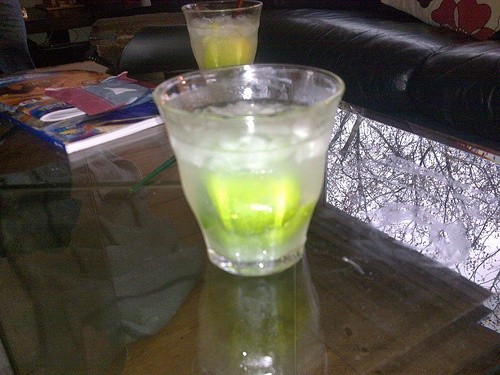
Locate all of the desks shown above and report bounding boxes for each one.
[2,108,499,375]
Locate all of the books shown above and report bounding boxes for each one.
[0,59,172,155]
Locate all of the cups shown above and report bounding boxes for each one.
[150,63,345,277]
[181,1,263,71]
